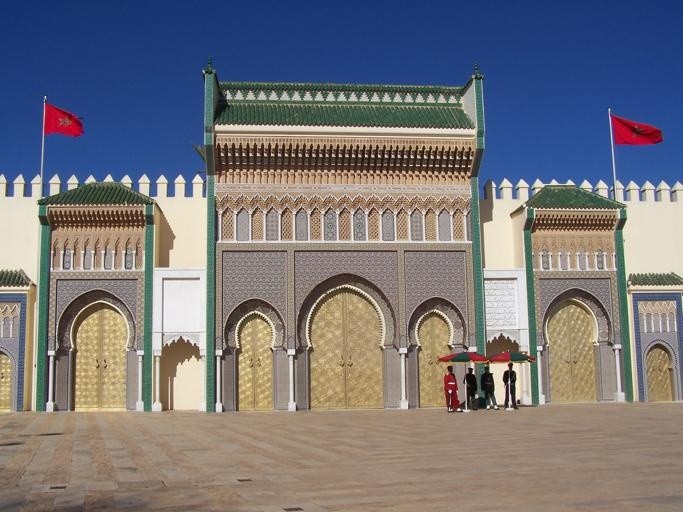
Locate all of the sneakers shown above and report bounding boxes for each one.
[446,406,519,412]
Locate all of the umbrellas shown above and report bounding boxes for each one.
[436,348,489,409]
[486,349,537,407]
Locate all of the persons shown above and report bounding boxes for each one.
[462,367,478,410]
[502,362,518,410]
[443,365,463,412]
[480,366,499,410]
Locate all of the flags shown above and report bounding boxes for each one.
[40,100,84,138]
[609,113,665,146]
